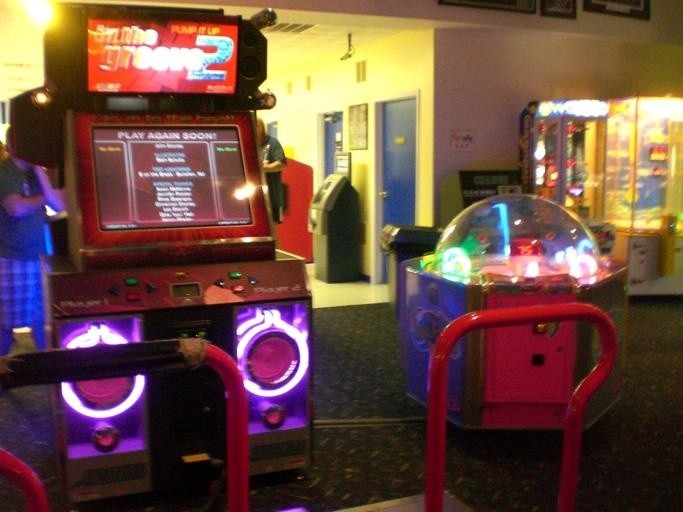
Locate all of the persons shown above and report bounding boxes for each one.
[0,125,64,354]
[255,118,287,239]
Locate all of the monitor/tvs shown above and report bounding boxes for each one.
[76,113,270,248]
[308,173,347,211]
